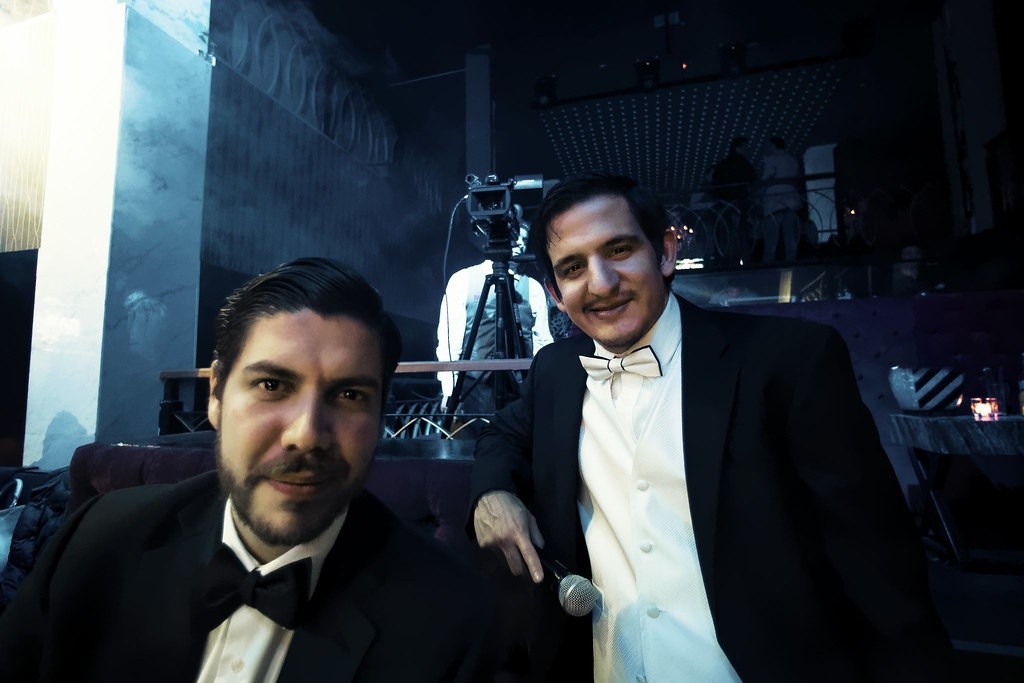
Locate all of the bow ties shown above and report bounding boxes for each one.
[578,346,663,380]
[181,542,312,631]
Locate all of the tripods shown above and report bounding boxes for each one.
[441,247,531,440]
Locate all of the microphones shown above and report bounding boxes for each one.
[531,544,596,616]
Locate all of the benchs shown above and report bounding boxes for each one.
[567,289,1024,505]
[66,444,533,566]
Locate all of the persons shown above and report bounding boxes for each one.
[462,170,954,683]
[710,134,801,271]
[0,257,505,683]
[435,219,554,442]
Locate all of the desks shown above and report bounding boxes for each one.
[887,414,1024,569]
